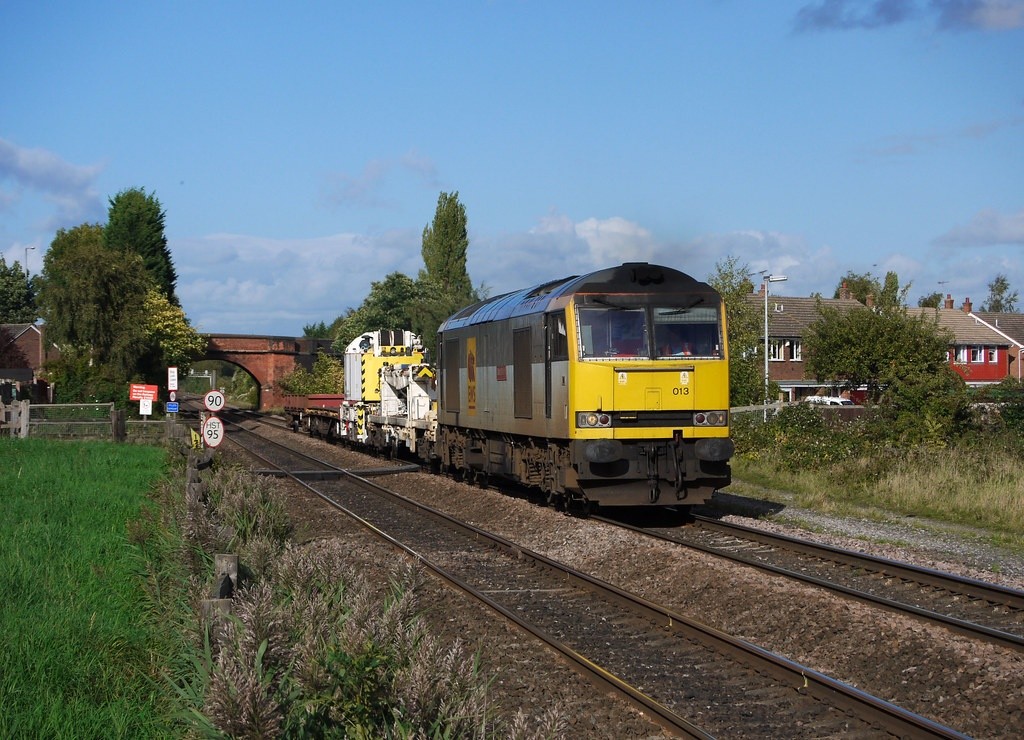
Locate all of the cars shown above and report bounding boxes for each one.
[805,395,854,406]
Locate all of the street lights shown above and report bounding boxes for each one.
[764,274,787,403]
[25,246,35,286]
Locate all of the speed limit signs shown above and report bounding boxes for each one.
[202,417,225,449]
[204,390,225,412]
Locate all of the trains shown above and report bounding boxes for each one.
[280,262,734,518]
